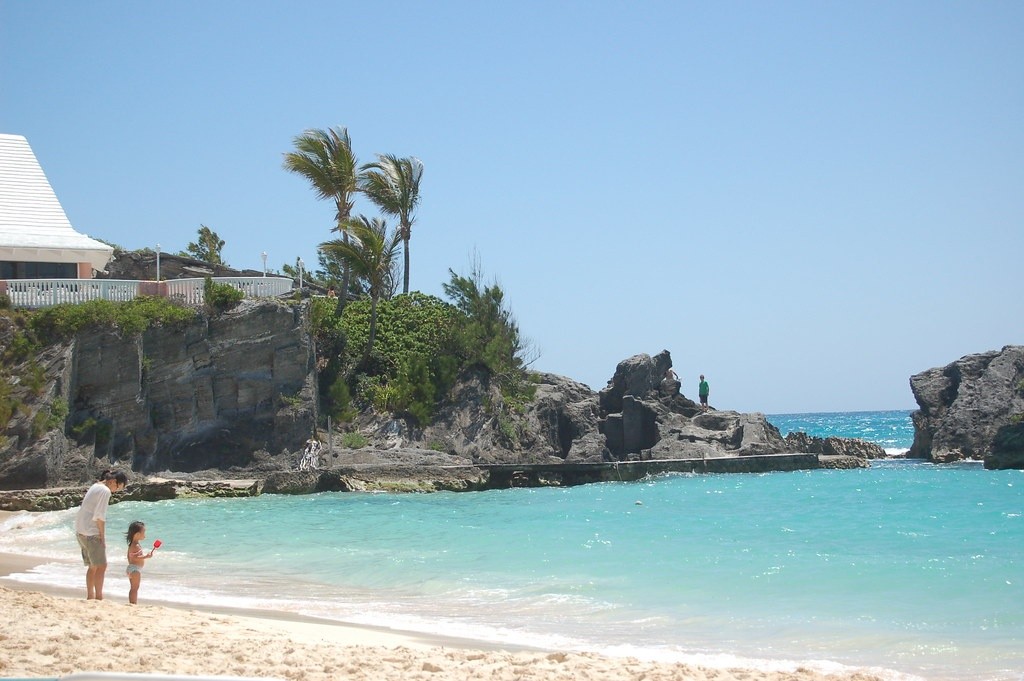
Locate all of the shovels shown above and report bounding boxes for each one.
[150,540,161,554]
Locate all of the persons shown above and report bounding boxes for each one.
[698,375,710,412]
[124,521,155,605]
[73,467,128,600]
[668,368,681,392]
